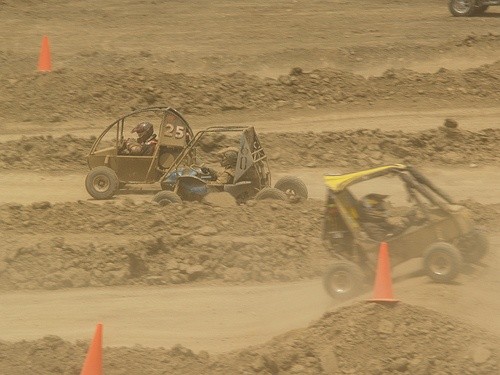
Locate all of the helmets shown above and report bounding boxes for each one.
[132,122,153,143]
[219,147,238,166]
[359,193,389,218]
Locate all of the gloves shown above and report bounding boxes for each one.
[121,136,127,147]
[201,167,208,173]
[401,209,416,225]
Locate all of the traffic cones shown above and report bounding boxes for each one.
[364,241,401,305]
[35,35,56,73]
[78,324,107,375]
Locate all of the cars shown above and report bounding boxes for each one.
[448,0,500,17]
[320,164,488,299]
[151,124,309,208]
[84,105,197,201]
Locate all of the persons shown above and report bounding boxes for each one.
[203,147,239,184]
[357,192,401,241]
[119,121,157,157]
